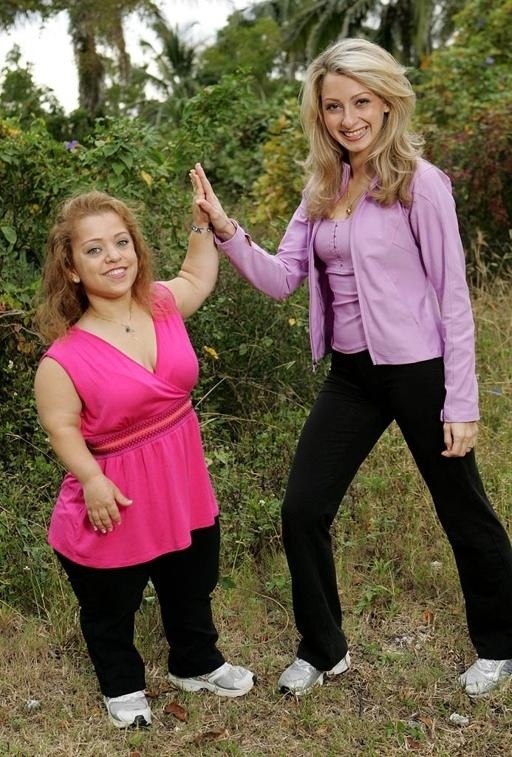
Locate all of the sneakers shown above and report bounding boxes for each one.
[104,690,152,728]
[459,658,512,698]
[278,652,351,696]
[168,662,256,698]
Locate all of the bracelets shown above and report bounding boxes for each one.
[190,224,211,234]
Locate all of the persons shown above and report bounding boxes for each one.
[33,167,257,729]
[195,38,512,699]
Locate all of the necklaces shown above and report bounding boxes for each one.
[346,188,364,216]
[89,299,133,332]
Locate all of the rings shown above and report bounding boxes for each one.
[468,445,474,448]
